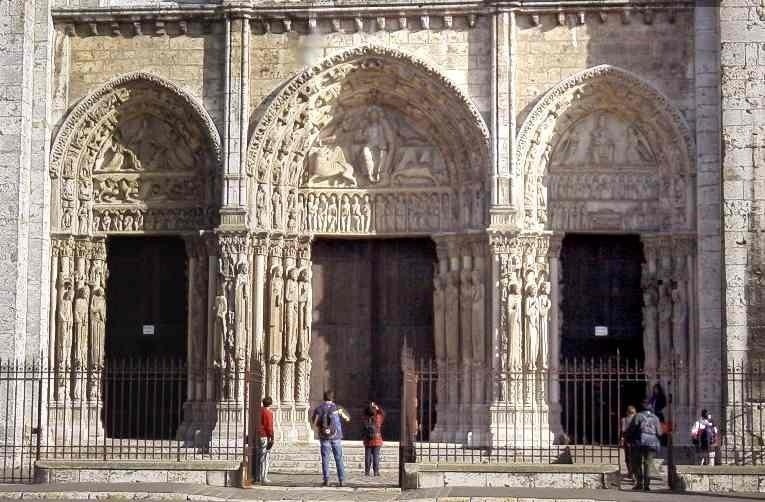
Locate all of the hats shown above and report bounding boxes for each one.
[641,399,652,410]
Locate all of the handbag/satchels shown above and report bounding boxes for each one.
[618,436,625,449]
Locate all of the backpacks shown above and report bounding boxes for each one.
[317,405,339,440]
[363,416,377,440]
[637,413,658,449]
[701,422,717,448]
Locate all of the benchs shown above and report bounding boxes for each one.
[32,458,246,488]
[674,463,765,494]
[404,460,620,489]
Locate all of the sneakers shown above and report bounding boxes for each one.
[322,480,344,487]
[364,472,380,477]
[254,477,271,485]
[626,473,651,493]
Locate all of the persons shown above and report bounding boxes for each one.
[311,390,353,486]
[620,383,720,491]
[361,400,385,477]
[256,395,275,484]
